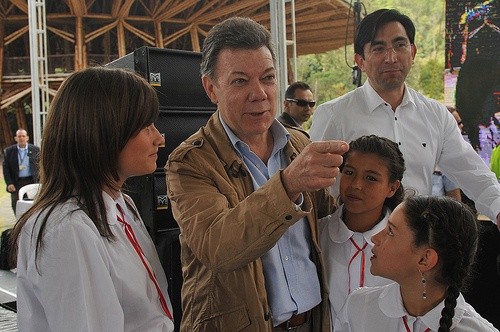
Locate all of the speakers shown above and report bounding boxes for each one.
[103,47,219,332]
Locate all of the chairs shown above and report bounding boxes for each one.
[18,184,41,201]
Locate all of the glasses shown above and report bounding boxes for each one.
[285,97,318,108]
[370,42,416,57]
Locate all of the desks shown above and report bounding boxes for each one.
[15,200,35,221]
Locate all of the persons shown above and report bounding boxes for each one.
[3,128,43,214]
[318,135,406,332]
[431,106,469,202]
[277,81,316,130]
[310,9,500,225]
[9,67,174,332]
[336,194,500,332]
[163,18,350,332]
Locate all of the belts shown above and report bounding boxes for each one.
[276,311,312,331]
